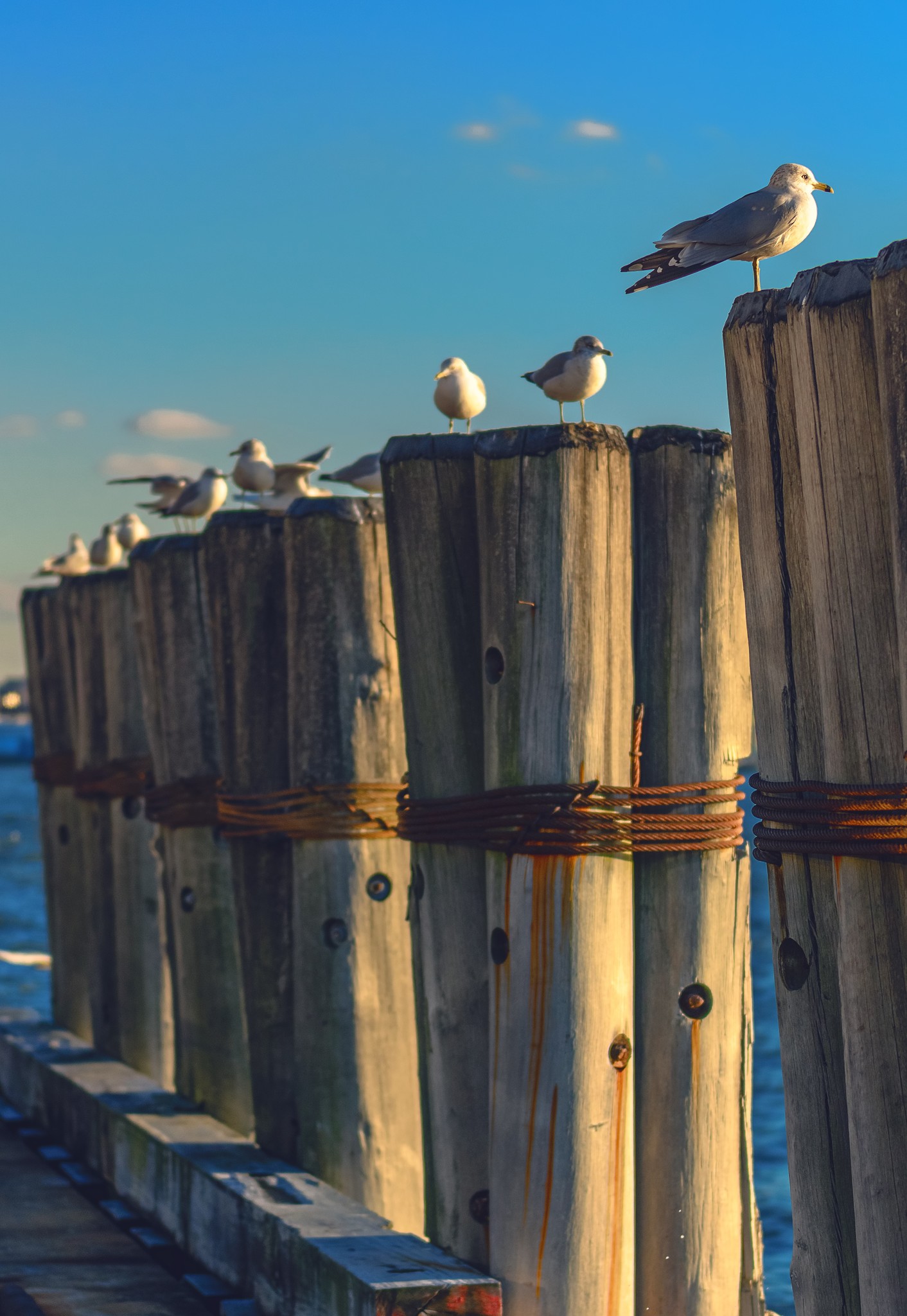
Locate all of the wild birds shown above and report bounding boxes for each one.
[520,335,613,424]
[232,446,334,510]
[229,438,276,511]
[105,474,194,534]
[433,357,487,435]
[159,466,232,536]
[318,450,384,496]
[34,512,149,577]
[620,163,834,296]
